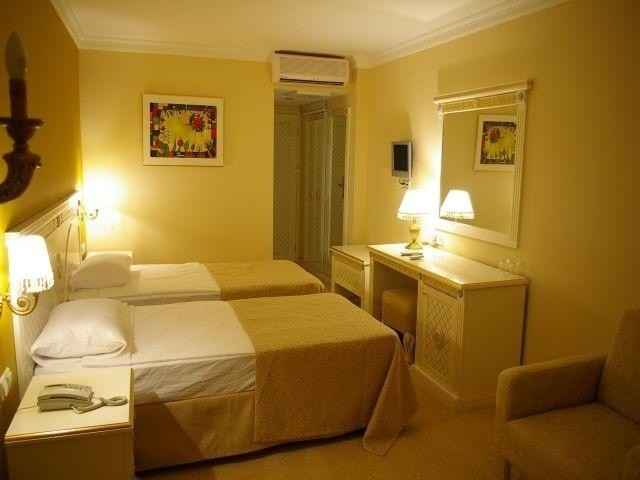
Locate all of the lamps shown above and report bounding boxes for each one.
[440,189,474,222]
[0,235,55,318]
[73,172,113,221]
[397,189,430,250]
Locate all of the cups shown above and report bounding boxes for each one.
[499,260,512,280]
[507,255,521,277]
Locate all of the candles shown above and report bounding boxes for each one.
[5,32,27,116]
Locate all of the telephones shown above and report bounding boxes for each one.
[36,384,97,414]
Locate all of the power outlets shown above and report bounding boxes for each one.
[1,369,12,399]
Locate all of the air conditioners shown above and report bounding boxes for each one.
[271,53,350,92]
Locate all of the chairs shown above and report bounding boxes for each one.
[496,308,640,480]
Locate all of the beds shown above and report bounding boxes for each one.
[66,260,328,306]
[34,292,419,470]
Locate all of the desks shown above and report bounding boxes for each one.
[366,241,528,412]
[330,242,369,311]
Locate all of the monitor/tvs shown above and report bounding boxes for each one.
[390,140,414,178]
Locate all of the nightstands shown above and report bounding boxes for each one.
[5,365,135,479]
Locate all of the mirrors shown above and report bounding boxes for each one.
[433,78,533,248]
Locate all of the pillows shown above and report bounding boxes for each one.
[68,254,132,288]
[30,298,131,359]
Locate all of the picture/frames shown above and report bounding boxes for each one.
[473,114,516,171]
[142,94,224,166]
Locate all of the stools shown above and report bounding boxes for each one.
[380,289,418,365]
